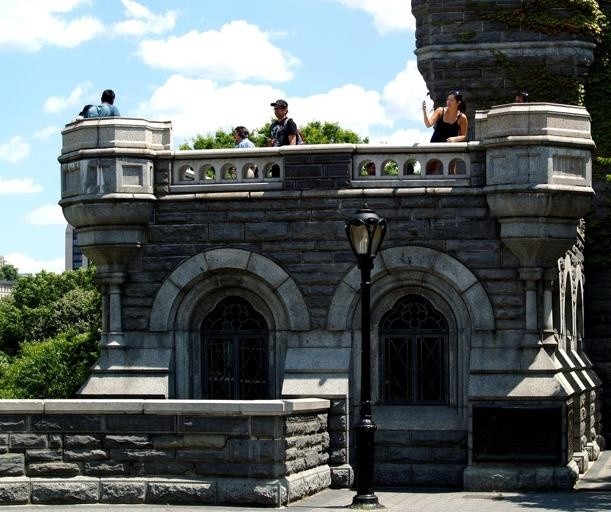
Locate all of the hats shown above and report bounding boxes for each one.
[271,100,288,107]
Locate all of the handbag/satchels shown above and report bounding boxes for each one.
[296,130,305,145]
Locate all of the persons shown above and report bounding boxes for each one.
[233,125,256,148]
[88,89,122,117]
[422,90,469,175]
[515,91,531,103]
[264,99,299,147]
[79,105,93,118]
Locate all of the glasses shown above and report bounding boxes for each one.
[449,90,459,95]
[520,91,529,97]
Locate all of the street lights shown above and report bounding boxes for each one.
[336,195,389,511]
